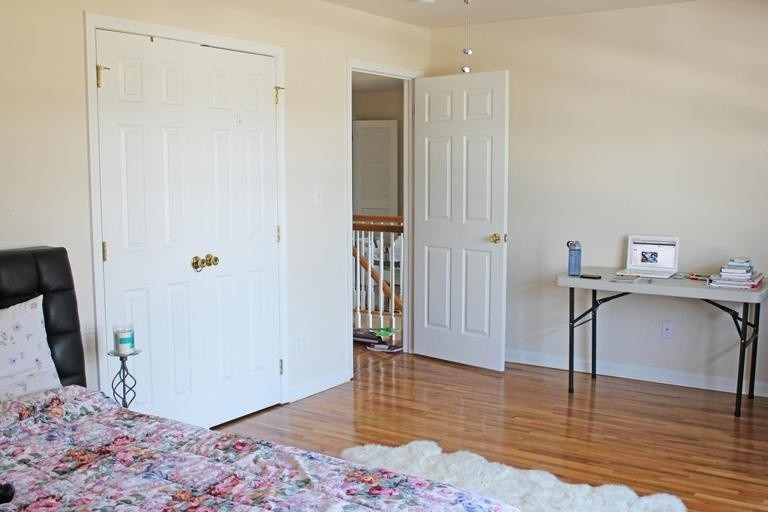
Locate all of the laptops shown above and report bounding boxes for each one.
[616,234,680,279]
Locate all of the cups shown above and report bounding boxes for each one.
[113,327,135,355]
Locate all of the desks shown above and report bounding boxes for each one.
[556,266,768,416]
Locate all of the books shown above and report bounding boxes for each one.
[609,275,640,283]
[354,329,403,353]
[708,256,764,290]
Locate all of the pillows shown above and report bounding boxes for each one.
[0,292,63,401]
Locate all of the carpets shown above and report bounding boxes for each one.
[340,440,687,512]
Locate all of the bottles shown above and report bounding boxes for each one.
[567,240,582,276]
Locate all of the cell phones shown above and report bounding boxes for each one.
[580,274,601,279]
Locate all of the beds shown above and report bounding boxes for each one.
[0,246,516,512]
